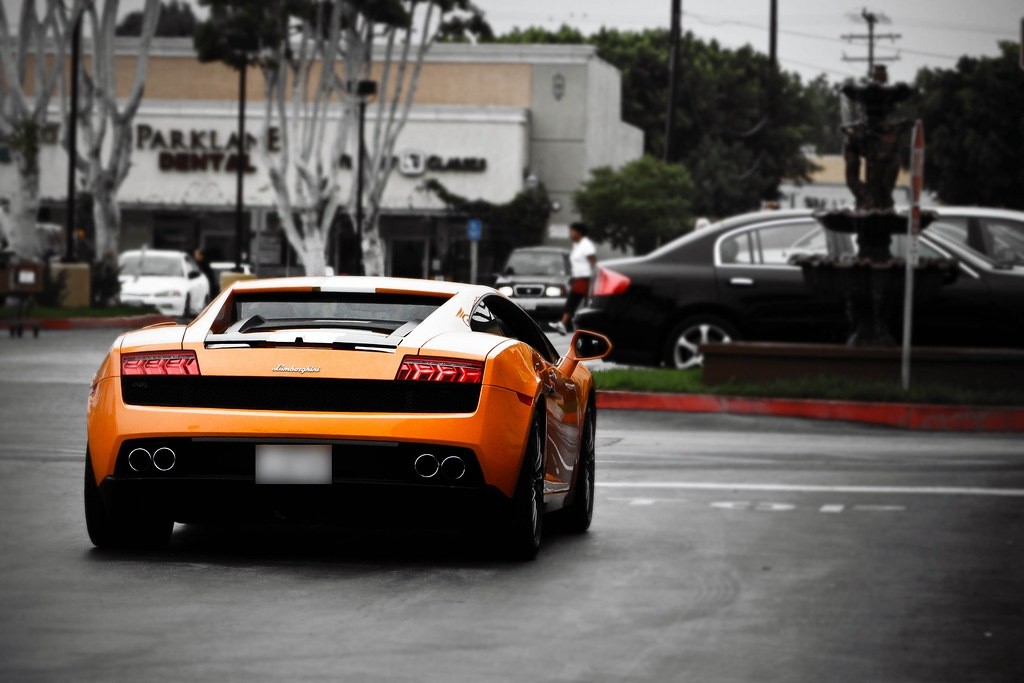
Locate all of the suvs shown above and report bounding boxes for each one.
[494,246,573,330]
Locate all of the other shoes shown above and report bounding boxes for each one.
[549,321,566,335]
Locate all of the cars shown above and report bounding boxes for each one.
[105,249,211,324]
[570,208,1023,372]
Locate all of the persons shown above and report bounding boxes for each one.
[548,222,597,336]
[193,248,217,303]
[102,249,115,268]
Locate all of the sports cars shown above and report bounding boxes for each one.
[80,272,612,561]
